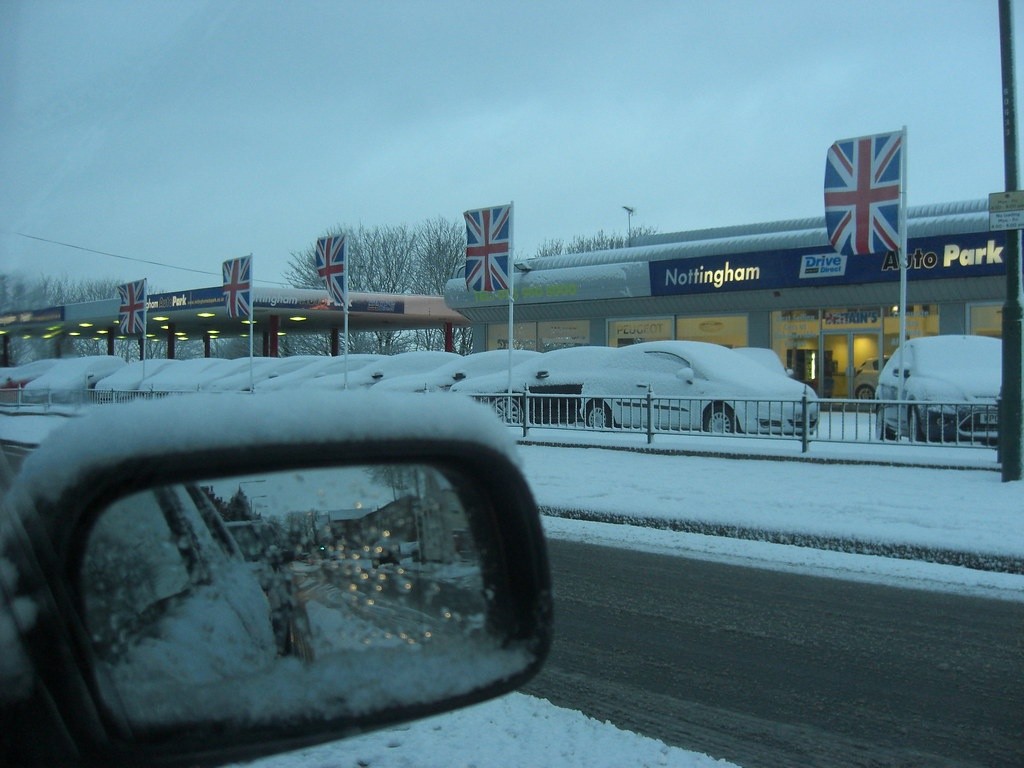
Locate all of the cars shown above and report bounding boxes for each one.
[446,346,613,427]
[23,350,547,403]
[6,359,70,388]
[228,520,310,658]
[854,355,894,406]
[578,340,821,439]
[873,335,1003,443]
[370,541,402,569]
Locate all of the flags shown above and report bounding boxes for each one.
[316,235,345,304]
[222,256,249,319]
[117,280,144,335]
[824,132,900,256]
[464,205,510,291]
